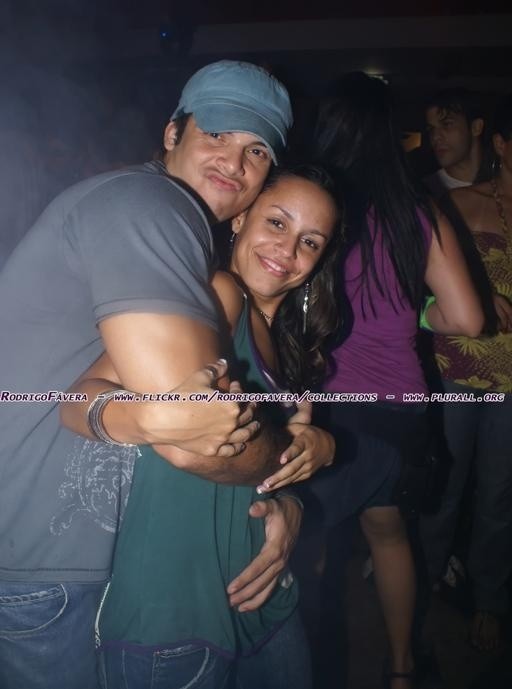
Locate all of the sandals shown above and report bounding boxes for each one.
[382,658,417,687]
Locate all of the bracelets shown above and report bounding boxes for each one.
[417,295,437,333]
[85,391,147,451]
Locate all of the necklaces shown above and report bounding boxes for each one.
[487,178,512,253]
[250,301,276,324]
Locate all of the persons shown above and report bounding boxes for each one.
[56,156,355,689]
[1,58,315,689]
[410,87,497,213]
[425,103,512,655]
[286,72,485,688]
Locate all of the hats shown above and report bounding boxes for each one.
[169,60,294,167]
